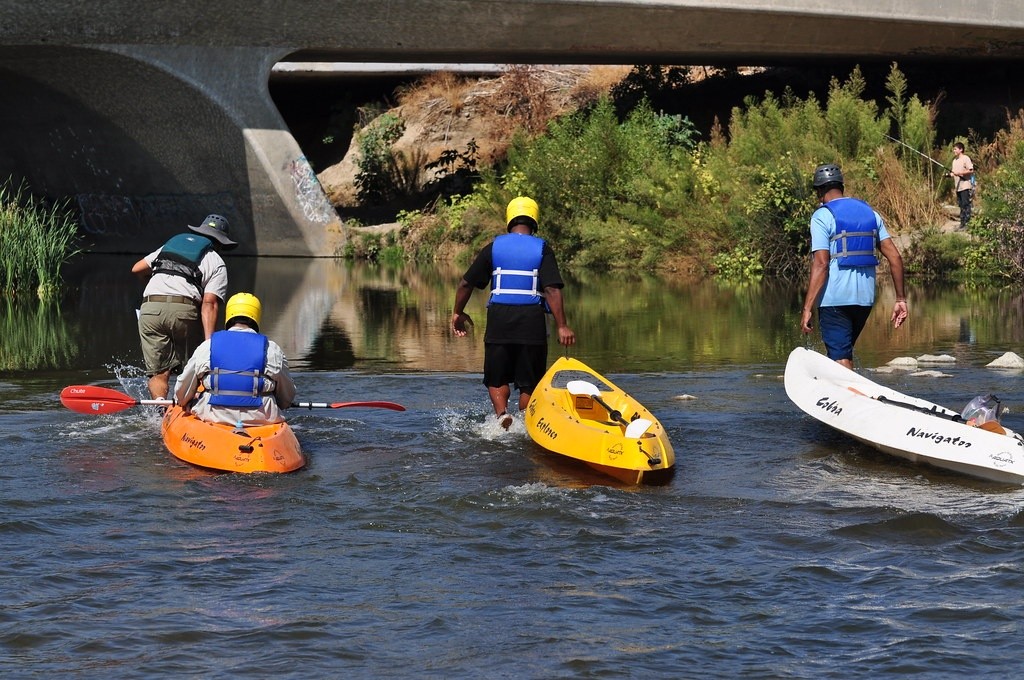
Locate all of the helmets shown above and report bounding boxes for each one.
[506,196,539,233]
[225,292,262,332]
[813,164,844,187]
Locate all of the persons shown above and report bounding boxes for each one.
[173,291,296,427]
[941,142,974,229]
[130,214,228,418]
[452,196,575,433]
[800,164,908,371]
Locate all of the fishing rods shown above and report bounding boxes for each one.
[877,128,961,180]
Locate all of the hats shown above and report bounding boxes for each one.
[188,214,239,251]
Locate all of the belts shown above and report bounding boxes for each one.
[141,295,196,304]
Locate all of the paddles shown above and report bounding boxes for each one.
[566,379,652,439]
[58,384,409,418]
[848,384,1008,437]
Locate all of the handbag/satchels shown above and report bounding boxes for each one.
[970,174,975,184]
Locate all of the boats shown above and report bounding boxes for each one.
[784,346,1024,485]
[525,356,675,485]
[160,380,307,474]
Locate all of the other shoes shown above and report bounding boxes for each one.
[497,407,513,430]
[154,405,167,417]
[954,224,967,232]
